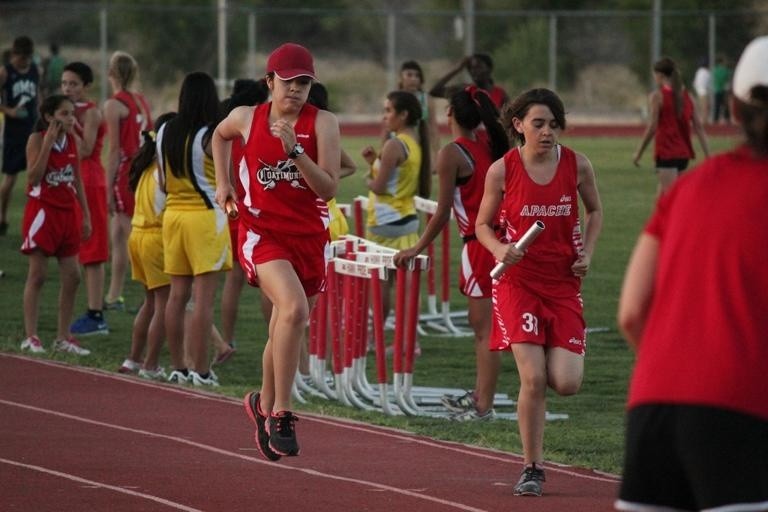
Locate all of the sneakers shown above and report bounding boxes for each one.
[53,336,92,355]
[264,410,301,457]
[68,315,110,338]
[446,410,497,423]
[211,342,234,366]
[102,294,139,315]
[119,360,223,388]
[512,464,545,496]
[441,392,476,411]
[0,222,9,235]
[244,390,281,461]
[19,335,44,353]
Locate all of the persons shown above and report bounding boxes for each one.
[360,51,512,424]
[691,60,713,124]
[0,35,271,391]
[208,41,342,464]
[709,56,731,124]
[476,89,605,499]
[631,57,711,202]
[618,35,767,510]
[305,83,356,241]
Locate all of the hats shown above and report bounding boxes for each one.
[732,34,768,104]
[266,43,319,82]
